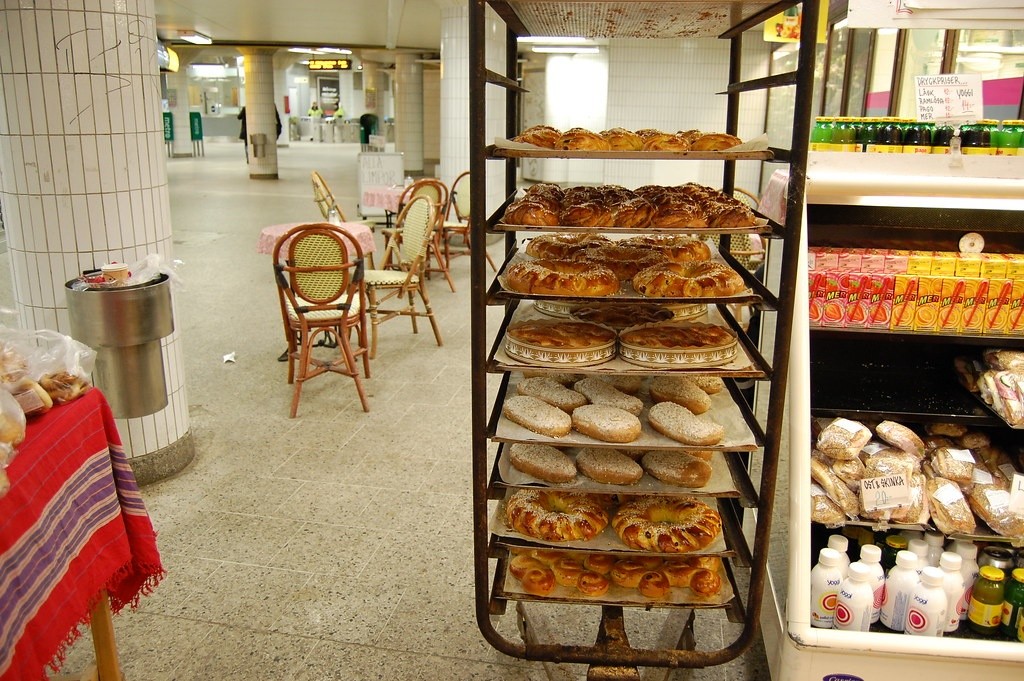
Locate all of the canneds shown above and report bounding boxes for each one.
[977,542,1024,588]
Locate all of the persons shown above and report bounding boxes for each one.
[333,104,344,120]
[308,102,323,118]
[238,107,249,163]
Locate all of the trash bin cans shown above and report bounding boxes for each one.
[63,268,174,420]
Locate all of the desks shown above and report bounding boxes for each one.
[0,387,168,681]
[256,221,376,360]
[363,186,415,228]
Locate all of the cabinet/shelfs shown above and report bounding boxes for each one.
[468,0,822,665]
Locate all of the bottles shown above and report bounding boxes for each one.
[404,176,414,189]
[329,211,340,227]
[809,116,1024,156]
[811,531,1024,643]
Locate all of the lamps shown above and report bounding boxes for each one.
[177,30,212,45]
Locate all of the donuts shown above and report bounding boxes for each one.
[506,491,722,552]
[506,232,746,297]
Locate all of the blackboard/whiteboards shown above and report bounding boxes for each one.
[357,152,405,217]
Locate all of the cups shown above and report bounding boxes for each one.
[101,263,129,286]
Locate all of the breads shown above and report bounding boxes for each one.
[509,438,715,488]
[503,299,739,368]
[508,547,721,598]
[504,182,759,228]
[811,344,1024,539]
[0,340,88,495]
[502,370,724,444]
[513,124,742,152]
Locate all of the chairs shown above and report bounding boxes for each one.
[339,178,449,359]
[312,172,374,269]
[426,172,497,279]
[273,223,371,419]
[381,180,456,296]
